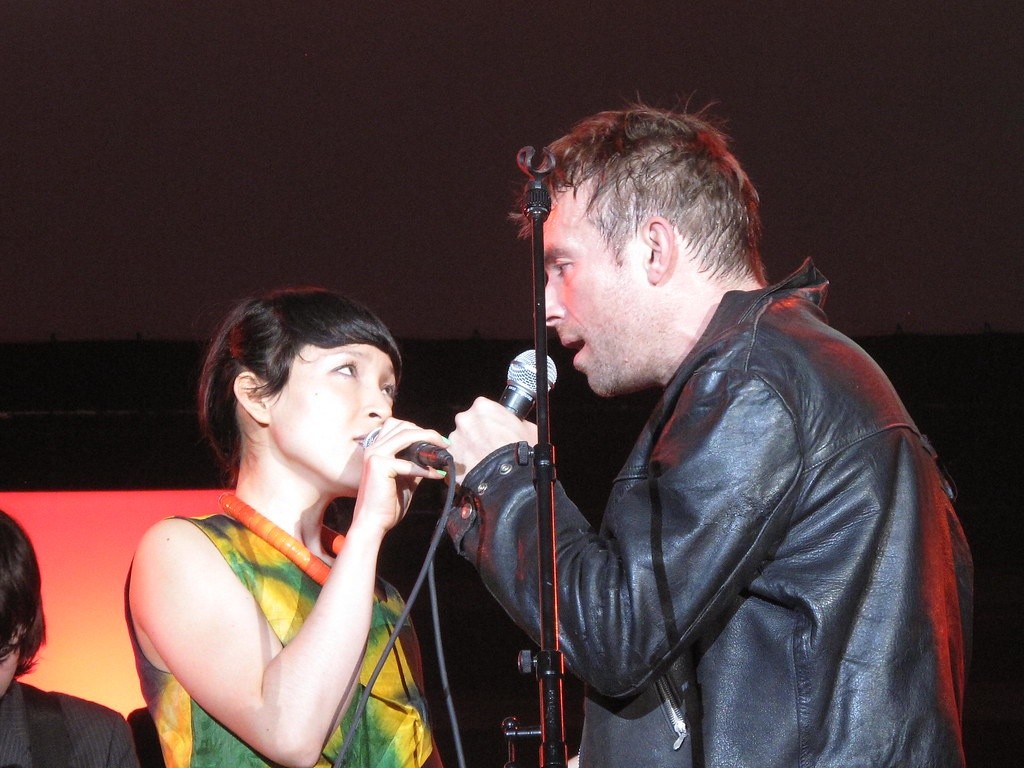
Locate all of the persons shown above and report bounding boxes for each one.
[124,284,451,768]
[437,103,972,768]
[1,504,140,767]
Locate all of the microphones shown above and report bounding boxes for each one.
[362,425,452,469]
[496,348,558,423]
